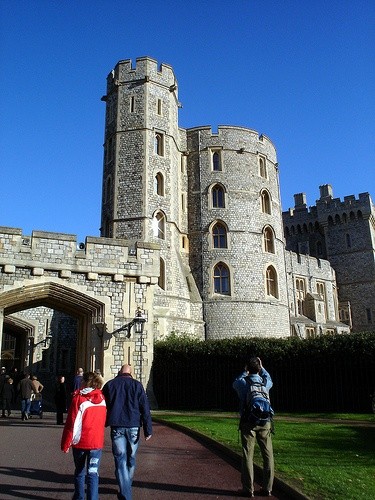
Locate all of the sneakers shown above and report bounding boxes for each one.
[237,488,273,499]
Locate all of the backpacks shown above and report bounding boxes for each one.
[242,382,274,422]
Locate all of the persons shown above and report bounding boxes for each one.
[101,364,153,500]
[232,357,276,498]
[60,372,106,500]
[0,367,85,424]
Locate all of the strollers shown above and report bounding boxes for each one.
[29,392,43,420]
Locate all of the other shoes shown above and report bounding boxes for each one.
[1,412,11,418]
[21,413,29,421]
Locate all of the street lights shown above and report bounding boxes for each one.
[127,307,145,339]
